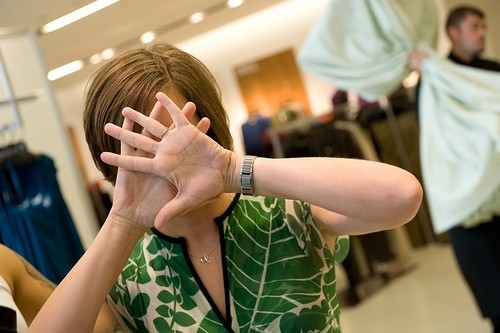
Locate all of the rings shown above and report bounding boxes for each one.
[160,128,169,138]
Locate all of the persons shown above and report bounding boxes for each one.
[410,5,500,333]
[26,42,424,333]
[0,244,57,333]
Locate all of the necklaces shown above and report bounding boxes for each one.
[189,238,220,263]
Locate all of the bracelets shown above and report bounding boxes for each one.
[240,155,256,196]
